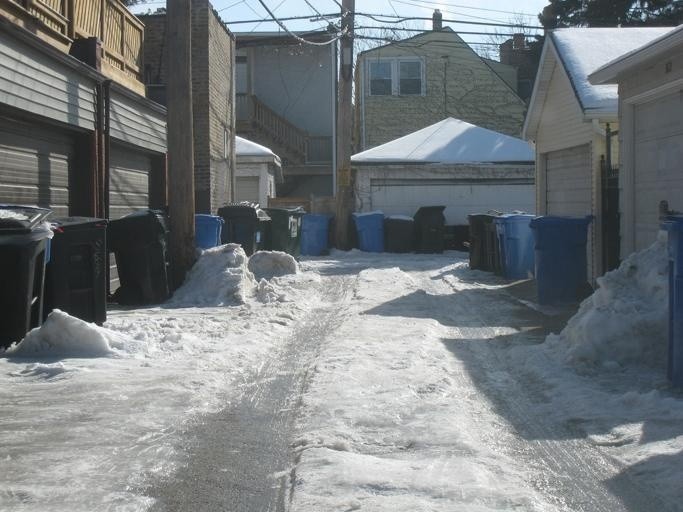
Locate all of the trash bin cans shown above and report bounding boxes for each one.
[300,213,335,256]
[0,205,55,347]
[493,214,536,279]
[385,215,415,252]
[218,201,272,256]
[260,206,307,260]
[352,211,385,251]
[659,213,683,396]
[195,214,225,248]
[467,211,497,272]
[107,209,177,306]
[415,206,447,254]
[528,214,595,304]
[44,217,107,325]
